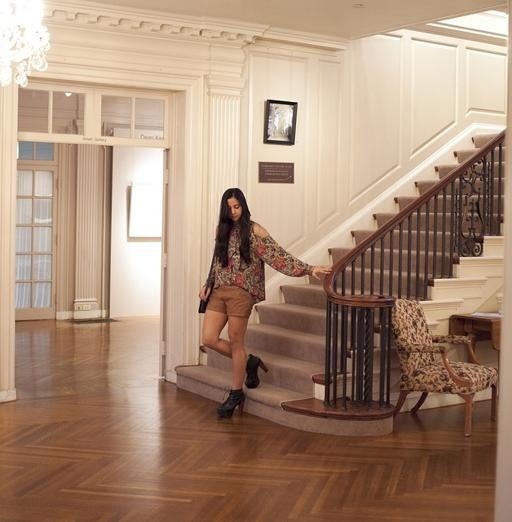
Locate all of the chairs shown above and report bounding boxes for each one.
[388,297,499,438]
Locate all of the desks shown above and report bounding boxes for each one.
[447,311,500,421]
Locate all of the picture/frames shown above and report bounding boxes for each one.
[264,98,298,145]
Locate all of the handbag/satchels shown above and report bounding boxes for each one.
[198,299,209,314]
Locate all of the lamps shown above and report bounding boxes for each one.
[1,1,53,89]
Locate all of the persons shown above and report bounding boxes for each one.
[198,188,334,418]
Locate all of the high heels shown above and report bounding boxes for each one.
[245,353,269,389]
[217,389,245,418]
